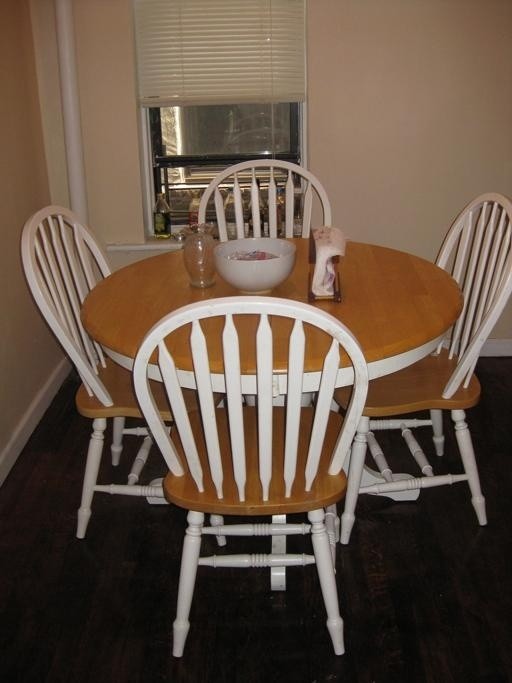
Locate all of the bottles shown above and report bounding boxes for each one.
[183,180,284,287]
[152,193,171,239]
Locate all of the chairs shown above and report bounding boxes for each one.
[128,289,374,654]
[196,157,331,242]
[18,201,227,539]
[331,184,512,545]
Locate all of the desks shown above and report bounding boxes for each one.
[81,233,466,588]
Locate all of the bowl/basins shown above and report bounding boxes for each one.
[213,237,295,295]
